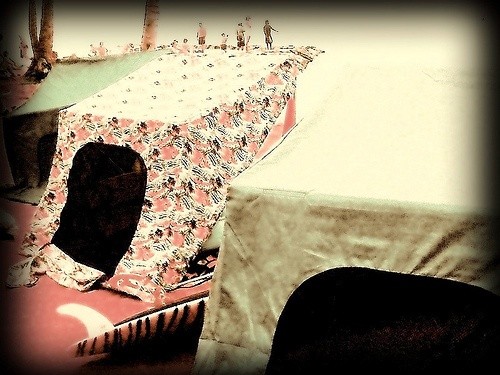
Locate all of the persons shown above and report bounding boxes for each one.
[235,22,246,51]
[262,19,279,50]
[14,30,192,61]
[242,15,254,51]
[220,32,231,50]
[195,22,207,46]
